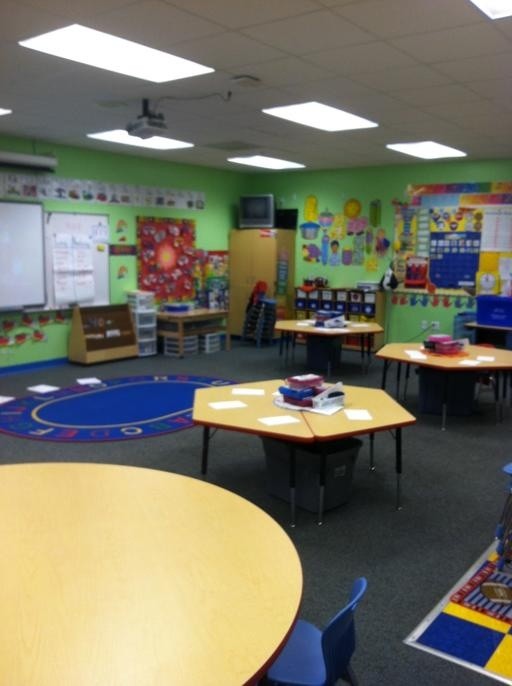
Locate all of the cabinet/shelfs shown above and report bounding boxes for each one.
[296,286,386,352]
[69,305,139,365]
[230,228,295,341]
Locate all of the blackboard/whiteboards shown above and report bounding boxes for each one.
[22,211,112,314]
[0,198,47,313]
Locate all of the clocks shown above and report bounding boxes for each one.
[475,271,501,295]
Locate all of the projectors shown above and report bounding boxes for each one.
[126,116,168,139]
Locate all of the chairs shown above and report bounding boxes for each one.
[242,281,277,349]
[269,577,369,686]
[496,462,512,570]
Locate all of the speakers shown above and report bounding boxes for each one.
[275,209,298,229]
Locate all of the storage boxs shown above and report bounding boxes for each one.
[417,370,480,417]
[306,336,341,369]
[476,296,512,327]
[128,290,158,357]
[259,438,363,511]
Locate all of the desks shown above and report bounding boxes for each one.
[156,308,231,358]
[192,381,417,529]
[0,462,305,685]
[275,320,384,370]
[375,343,512,432]
[464,322,512,332]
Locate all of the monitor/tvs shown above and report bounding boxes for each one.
[238,194,274,228]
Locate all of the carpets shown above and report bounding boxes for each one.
[0,375,237,443]
